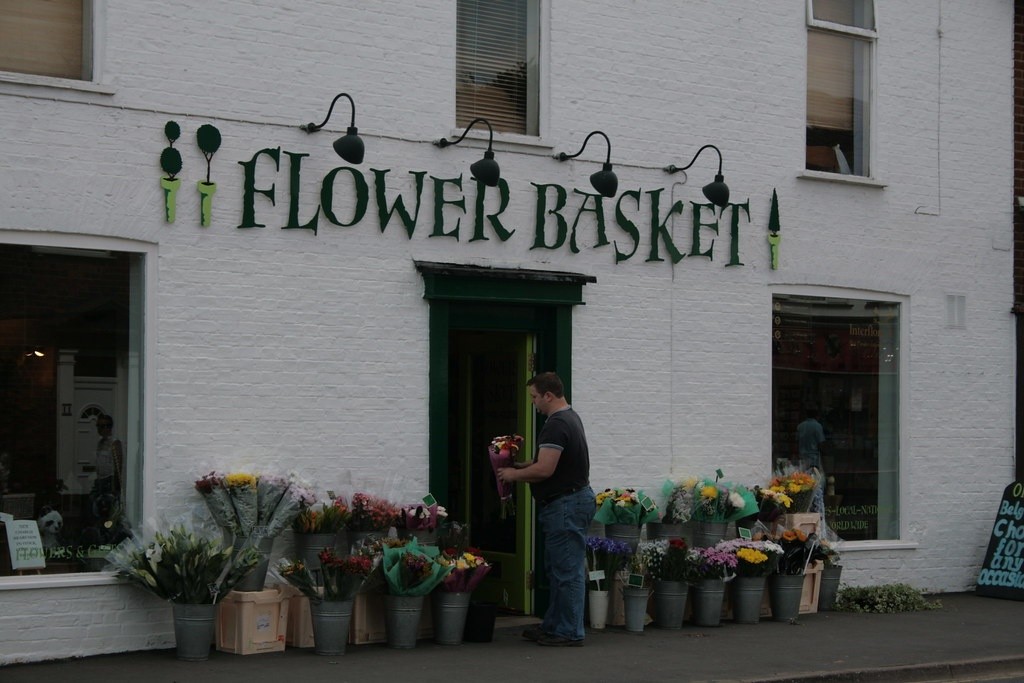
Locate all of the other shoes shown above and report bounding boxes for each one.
[538,633,584,646]
[523,626,550,640]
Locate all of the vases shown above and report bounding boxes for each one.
[280,583,368,647]
[692,520,729,549]
[603,523,642,554]
[431,593,470,645]
[310,600,356,655]
[622,588,651,631]
[462,562,502,644]
[381,594,423,648]
[607,559,823,626]
[217,585,289,654]
[367,586,435,643]
[819,566,842,610]
[588,590,611,629]
[652,581,690,629]
[730,578,765,623]
[172,603,219,660]
[770,512,821,556]
[769,574,806,621]
[689,580,727,626]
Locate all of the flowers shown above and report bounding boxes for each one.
[586,459,843,591]
[100,433,525,604]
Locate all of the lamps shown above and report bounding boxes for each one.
[559,131,619,199]
[663,145,730,208]
[306,92,365,164]
[440,118,500,187]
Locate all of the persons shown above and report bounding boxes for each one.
[94,414,124,532]
[497,371,598,647]
[795,400,828,506]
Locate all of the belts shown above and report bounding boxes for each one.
[543,484,590,506]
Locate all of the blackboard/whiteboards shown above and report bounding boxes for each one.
[973,479,1024,602]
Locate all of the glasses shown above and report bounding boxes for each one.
[96,424,110,428]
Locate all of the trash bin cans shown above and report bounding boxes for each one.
[3,492,36,520]
